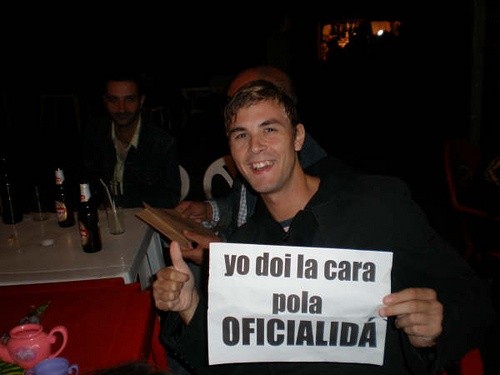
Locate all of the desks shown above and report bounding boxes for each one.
[0,205,170,374]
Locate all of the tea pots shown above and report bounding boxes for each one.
[0,324,67,370]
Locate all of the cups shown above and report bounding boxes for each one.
[26,181,47,222]
[103,179,126,235]
[24,357,79,375]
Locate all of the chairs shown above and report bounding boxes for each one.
[443,137,500,262]
[203,154,238,202]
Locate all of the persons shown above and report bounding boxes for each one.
[81,75,193,208]
[175,52,339,296]
[153,80,496,375]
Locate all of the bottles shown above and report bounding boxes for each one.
[0,153,23,224]
[52,166,75,228]
[77,178,102,255]
